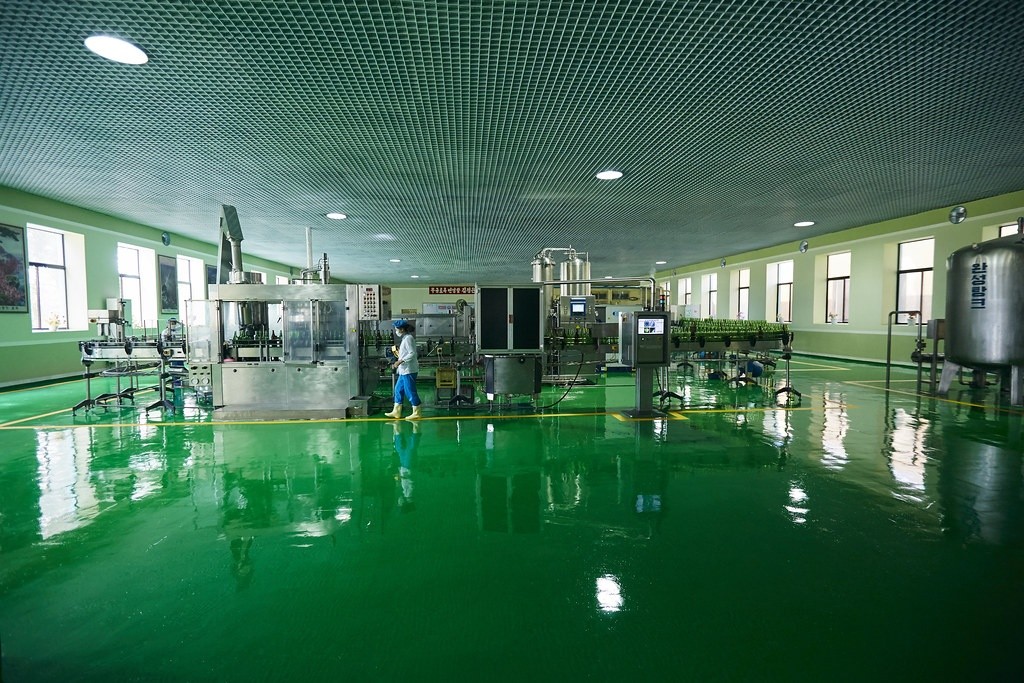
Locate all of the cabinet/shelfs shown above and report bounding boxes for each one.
[475,282,544,354]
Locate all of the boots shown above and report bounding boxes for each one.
[384,403,402,419]
[385,420,401,435]
[405,405,421,420]
[406,419,420,434]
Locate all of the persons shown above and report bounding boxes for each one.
[729,355,764,386]
[161,318,185,343]
[385,320,421,419]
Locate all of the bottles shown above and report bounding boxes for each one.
[544,328,618,345]
[427,337,432,353]
[359,329,395,346]
[670,317,790,341]
[232,330,282,348]
[290,330,345,347]
[439,336,443,350]
[450,336,454,353]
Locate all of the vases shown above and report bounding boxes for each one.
[49,324,57,331]
[831,319,837,324]
[907,319,916,326]
[779,317,784,323]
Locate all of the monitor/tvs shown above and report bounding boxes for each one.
[637,319,664,334]
[573,305,584,312]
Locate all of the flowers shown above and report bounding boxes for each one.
[828,312,838,319]
[909,312,917,319]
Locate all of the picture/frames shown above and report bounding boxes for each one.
[0,223,29,313]
[205,264,217,300]
[158,254,179,314]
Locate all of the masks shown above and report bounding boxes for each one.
[396,330,404,337]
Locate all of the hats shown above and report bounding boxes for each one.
[392,320,408,328]
